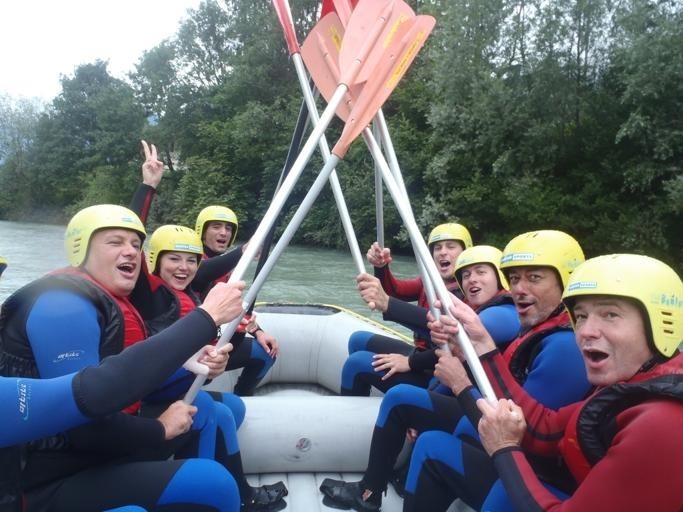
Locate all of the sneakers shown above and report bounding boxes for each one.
[319,478,381,511]
[239,480,289,510]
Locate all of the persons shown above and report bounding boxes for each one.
[0,202,242,511]
[127,138,287,511]
[430,253,683,511]
[402,228,593,512]
[194,205,279,396]
[317,244,521,511]
[339,222,478,396]
[0,255,248,446]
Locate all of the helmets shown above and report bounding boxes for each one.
[560,253,683,359]
[428,222,472,251]
[146,224,204,275]
[63,203,148,267]
[499,229,586,304]
[452,244,511,298]
[195,204,239,250]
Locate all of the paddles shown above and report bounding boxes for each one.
[182,13,438,409]
[227,1,417,299]
[298,10,502,408]
[274,0,454,361]
[244,2,359,316]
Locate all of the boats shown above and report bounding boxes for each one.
[191,301,480,511]
